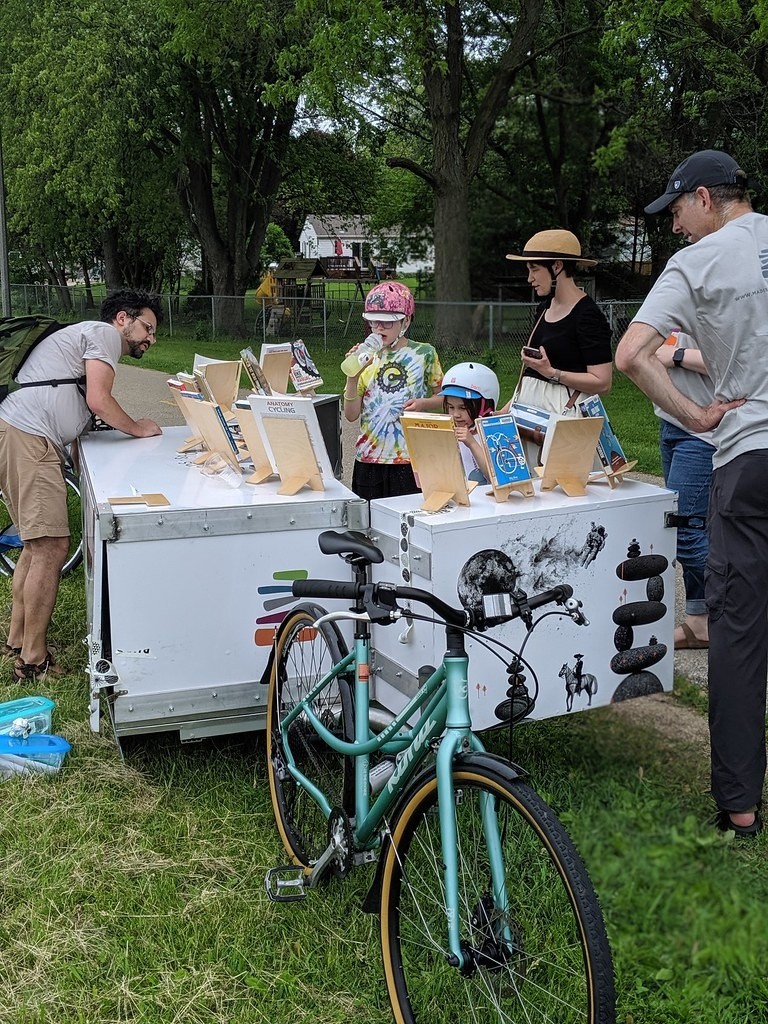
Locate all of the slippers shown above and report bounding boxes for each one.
[674,623,708,649]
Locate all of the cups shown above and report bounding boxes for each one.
[203,451,242,487]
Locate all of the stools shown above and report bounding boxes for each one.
[0,522,24,577]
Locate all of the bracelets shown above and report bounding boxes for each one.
[343,391,359,401]
[549,369,561,385]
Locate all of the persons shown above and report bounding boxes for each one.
[341,280,445,530]
[0,289,163,688]
[650,330,718,650]
[491,229,616,481]
[614,149,768,837]
[436,361,507,493]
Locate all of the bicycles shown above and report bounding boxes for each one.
[0,472,87,580]
[256,530,618,1024]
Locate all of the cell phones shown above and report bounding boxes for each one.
[522,345,542,360]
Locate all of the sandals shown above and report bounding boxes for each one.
[2,643,58,662]
[13,651,74,686]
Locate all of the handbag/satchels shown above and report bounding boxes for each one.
[508,403,557,448]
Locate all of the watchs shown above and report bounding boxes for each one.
[672,347,687,369]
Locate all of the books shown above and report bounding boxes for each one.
[166,337,340,492]
[477,414,532,487]
[398,410,469,490]
[578,394,628,476]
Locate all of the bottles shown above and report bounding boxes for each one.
[340,333,384,377]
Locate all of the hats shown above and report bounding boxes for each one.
[506,230,598,267]
[644,150,748,216]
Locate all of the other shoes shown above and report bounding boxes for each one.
[723,810,763,838]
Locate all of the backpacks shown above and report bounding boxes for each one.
[0,314,86,403]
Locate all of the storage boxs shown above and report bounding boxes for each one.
[0,695,71,777]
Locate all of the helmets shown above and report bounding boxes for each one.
[441,362,499,412]
[365,282,415,317]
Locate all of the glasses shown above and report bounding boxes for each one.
[113,312,156,344]
[367,320,403,329]
